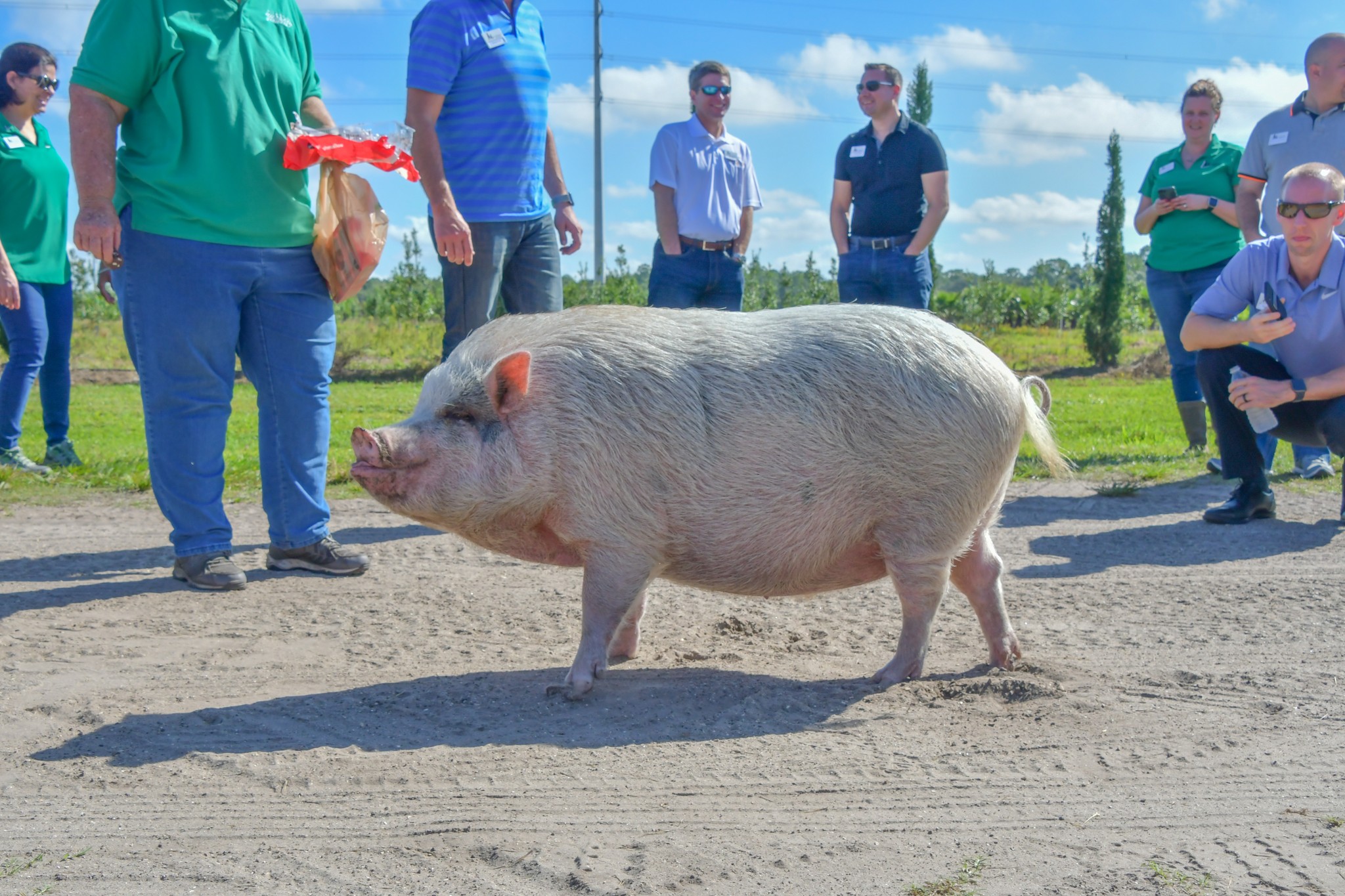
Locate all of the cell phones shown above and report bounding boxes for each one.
[1264,281,1287,323]
[1158,186,1177,202]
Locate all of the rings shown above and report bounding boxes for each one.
[1243,394,1248,402]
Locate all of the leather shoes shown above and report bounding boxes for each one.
[1202,483,1276,524]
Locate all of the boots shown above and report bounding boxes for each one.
[1177,400,1209,458]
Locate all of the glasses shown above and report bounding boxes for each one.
[1277,198,1345,219]
[857,81,895,94]
[695,85,731,95]
[17,72,59,91]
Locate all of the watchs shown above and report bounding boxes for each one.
[551,191,575,209]
[1290,377,1307,403]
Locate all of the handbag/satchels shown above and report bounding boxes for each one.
[312,159,389,305]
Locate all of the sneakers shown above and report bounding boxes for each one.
[0,446,53,478]
[265,533,371,577]
[1207,458,1273,476]
[173,549,248,591]
[43,440,84,467]
[1294,454,1335,479]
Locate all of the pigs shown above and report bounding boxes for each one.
[352,303,1076,698]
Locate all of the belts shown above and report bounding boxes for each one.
[850,235,913,250]
[678,235,734,251]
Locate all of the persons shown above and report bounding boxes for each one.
[69,1,368,593]
[1179,162,1345,526]
[1133,78,1245,456]
[646,60,763,312]
[405,0,583,368]
[829,63,950,310]
[1206,33,1345,480]
[0,41,85,478]
[95,260,116,304]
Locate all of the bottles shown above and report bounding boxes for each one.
[1230,365,1280,434]
